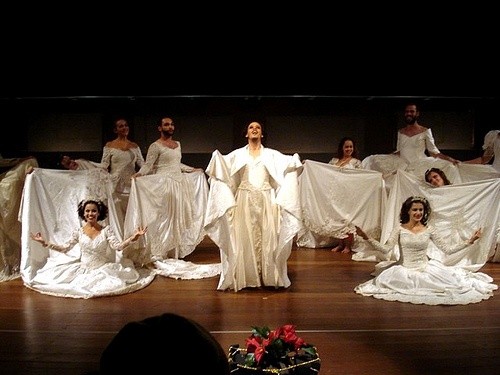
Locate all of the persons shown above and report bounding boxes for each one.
[366,103,466,188]
[394,163,500,262]
[302,137,383,254]
[25,151,110,175]
[0,153,33,275]
[129,116,210,279]
[29,197,149,293]
[90,117,144,262]
[354,197,482,294]
[211,120,305,292]
[456,130,500,184]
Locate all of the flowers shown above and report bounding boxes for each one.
[241,324,305,366]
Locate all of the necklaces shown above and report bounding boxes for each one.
[249,143,261,154]
